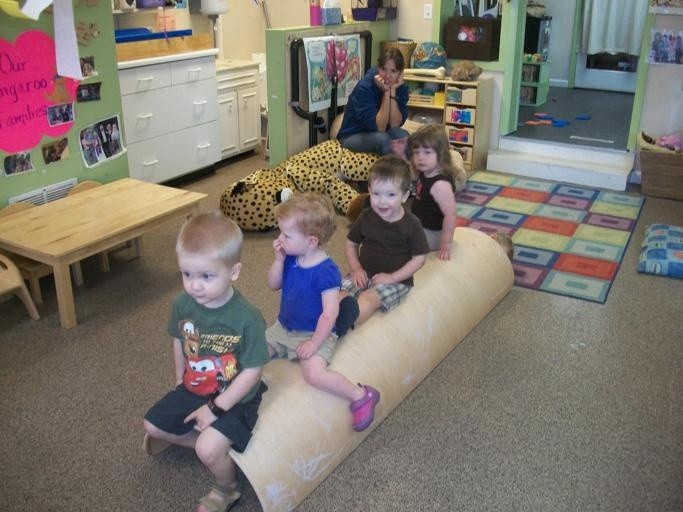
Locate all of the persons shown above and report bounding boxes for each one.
[487,230,514,265]
[263,194,378,433]
[81,124,120,166]
[335,47,409,157]
[80,57,94,78]
[650,31,683,64]
[142,214,269,512]
[405,125,455,262]
[336,156,430,339]
[48,104,71,125]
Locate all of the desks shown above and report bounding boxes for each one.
[0,178,209,330]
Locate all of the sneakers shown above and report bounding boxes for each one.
[350,383,380,432]
[336,296,360,336]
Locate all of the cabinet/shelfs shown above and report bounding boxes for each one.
[214,54,262,167]
[520,57,556,109]
[116,48,222,186]
[397,69,497,177]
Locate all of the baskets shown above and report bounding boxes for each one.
[381,38,416,69]
[639,147,683,201]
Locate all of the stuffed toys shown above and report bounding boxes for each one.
[220,141,381,234]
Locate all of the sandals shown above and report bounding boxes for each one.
[194,482,241,512]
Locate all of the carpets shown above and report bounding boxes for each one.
[434,165,646,308]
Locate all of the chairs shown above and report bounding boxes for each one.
[67,180,147,272]
[0,255,40,327]
[0,204,82,303]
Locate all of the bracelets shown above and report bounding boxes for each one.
[389,95,399,102]
[206,399,225,419]
